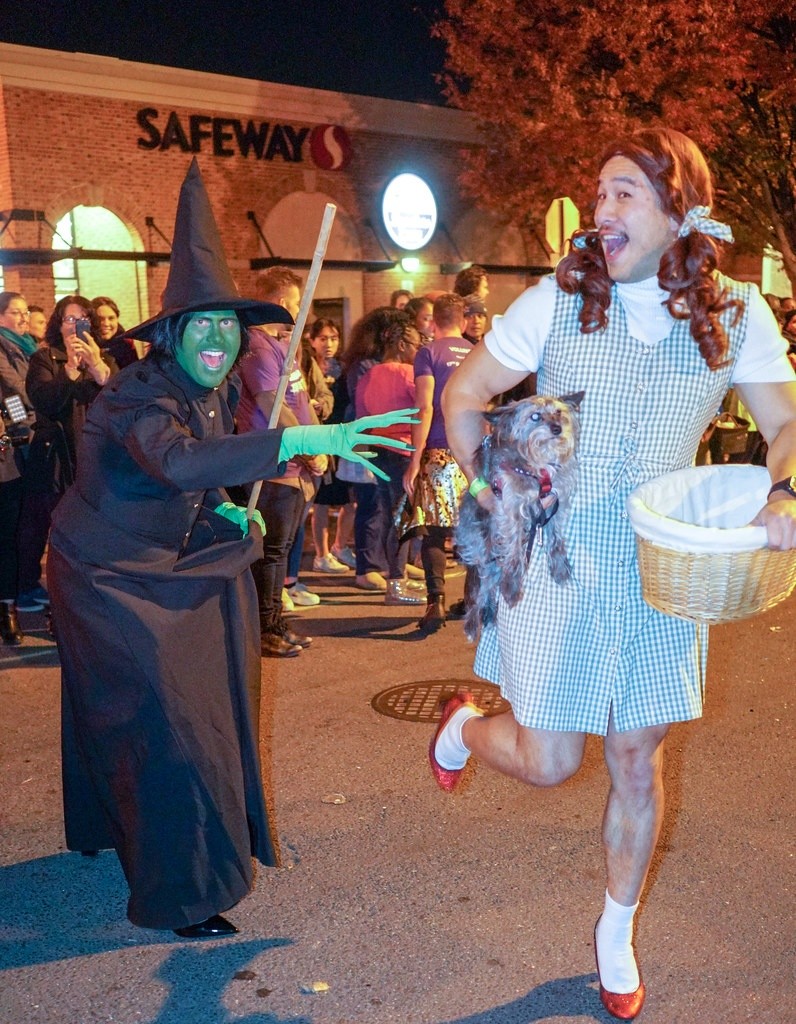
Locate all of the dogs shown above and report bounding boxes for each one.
[452,390,586,642]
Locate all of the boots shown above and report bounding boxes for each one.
[385,578,427,605]
[418,594,446,631]
[450,598,467,615]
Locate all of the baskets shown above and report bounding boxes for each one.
[708,412,751,454]
[625,463,796,626]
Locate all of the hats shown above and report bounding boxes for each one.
[114,154,296,342]
[464,302,488,318]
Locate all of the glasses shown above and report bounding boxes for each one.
[2,309,30,317]
[62,315,91,324]
[404,340,423,350]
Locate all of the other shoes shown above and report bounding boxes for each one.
[355,572,387,591]
[446,559,458,568]
[405,563,426,577]
[11,586,50,612]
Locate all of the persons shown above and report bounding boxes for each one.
[25,296,123,504]
[0,265,796,657]
[427,129,796,1020]
[43,307,421,939]
[232,265,329,656]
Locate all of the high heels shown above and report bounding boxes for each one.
[428,692,479,793]
[594,913,646,1021]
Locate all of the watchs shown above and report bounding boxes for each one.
[767,476,796,497]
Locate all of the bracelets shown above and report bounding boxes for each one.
[468,477,490,496]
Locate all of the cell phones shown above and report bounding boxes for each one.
[76,319,91,345]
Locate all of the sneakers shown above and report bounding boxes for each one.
[330,543,356,569]
[288,583,320,605]
[281,589,294,612]
[313,554,349,573]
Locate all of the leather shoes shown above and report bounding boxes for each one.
[285,629,315,648]
[172,914,240,938]
[0,601,24,643]
[260,633,302,658]
